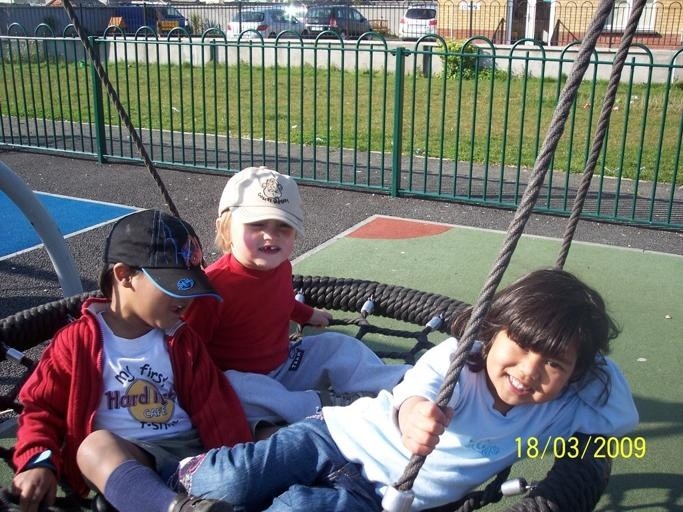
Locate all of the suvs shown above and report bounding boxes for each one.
[396,3,438,42]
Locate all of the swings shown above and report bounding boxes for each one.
[0,0,648,511]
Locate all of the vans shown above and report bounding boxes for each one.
[304,6,372,41]
[228,8,310,39]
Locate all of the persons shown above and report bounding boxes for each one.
[166,269,640,511]
[12,210,255,512]
[176,166,414,425]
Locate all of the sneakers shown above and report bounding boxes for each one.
[312,387,378,410]
[170,492,235,512]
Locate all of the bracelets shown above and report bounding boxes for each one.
[23,450,55,470]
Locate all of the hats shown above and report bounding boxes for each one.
[103,208,222,303]
[217,164,307,238]
